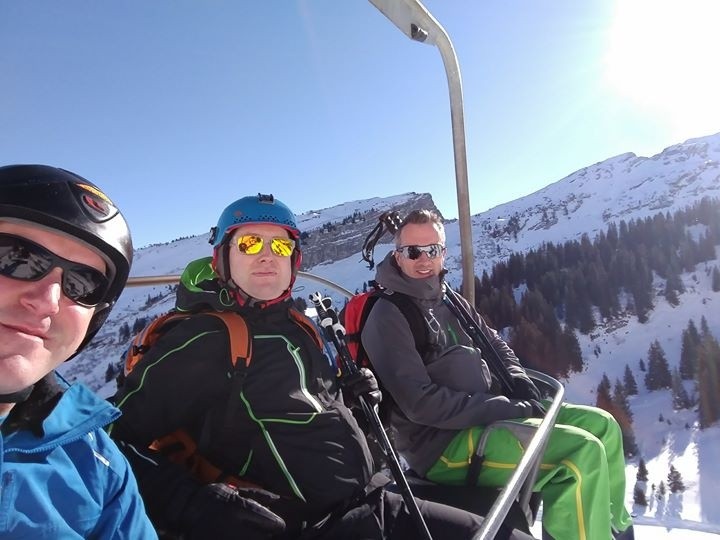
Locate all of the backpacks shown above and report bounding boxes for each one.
[335,290,430,427]
[124,310,323,488]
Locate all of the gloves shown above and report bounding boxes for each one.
[156,478,287,540]
[501,373,548,418]
[339,368,383,417]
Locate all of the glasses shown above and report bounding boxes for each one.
[0,231,110,308]
[227,233,297,258]
[397,244,447,260]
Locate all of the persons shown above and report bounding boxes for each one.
[359,208,636,540]
[107,194,532,540]
[1,164,159,540]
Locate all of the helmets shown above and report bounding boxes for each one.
[0,164,134,362]
[208,193,302,283]
